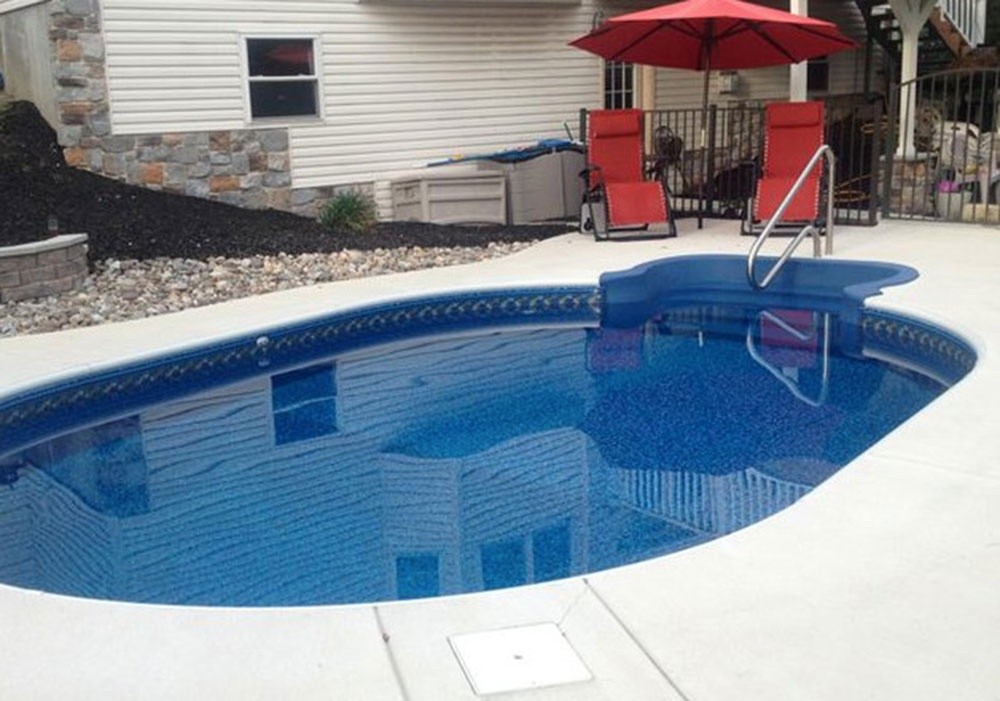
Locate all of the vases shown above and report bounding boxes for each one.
[934,192,963,218]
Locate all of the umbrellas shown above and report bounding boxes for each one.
[573,373,771,475]
[566,0,864,227]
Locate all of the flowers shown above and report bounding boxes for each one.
[936,179,960,192]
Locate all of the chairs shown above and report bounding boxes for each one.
[740,100,832,237]
[929,132,1000,216]
[932,117,996,161]
[577,106,678,241]
